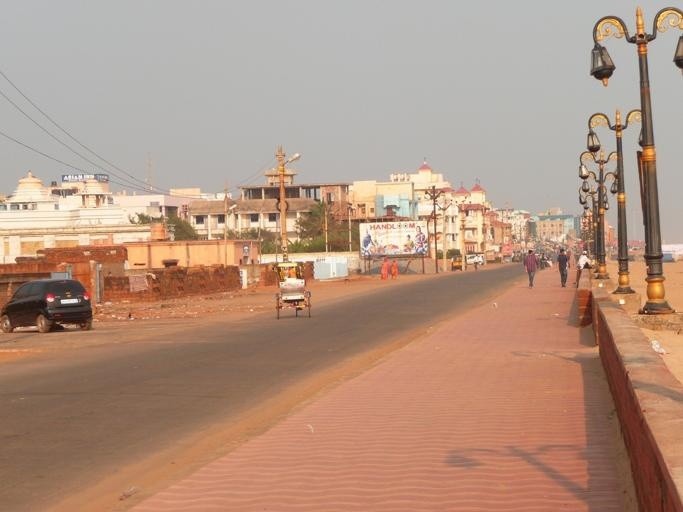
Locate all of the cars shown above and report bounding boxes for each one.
[502,251,559,269]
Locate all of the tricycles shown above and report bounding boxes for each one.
[274,262,311,321]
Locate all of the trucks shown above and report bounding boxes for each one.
[451,254,467,271]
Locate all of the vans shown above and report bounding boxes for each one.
[465,255,484,265]
[0,278,91,334]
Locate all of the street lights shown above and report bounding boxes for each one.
[590,4,682,315]
[275,145,301,262]
[324,202,334,253]
[587,109,642,293]
[346,204,356,252]
[453,200,471,271]
[577,149,617,278]
[223,187,237,270]
[501,210,564,248]
[431,200,455,272]
[423,185,445,274]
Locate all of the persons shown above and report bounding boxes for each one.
[576,250,591,288]
[388,259,398,279]
[473,254,478,270]
[521,250,538,288]
[402,235,412,252]
[412,225,425,254]
[380,257,389,279]
[556,248,568,287]
